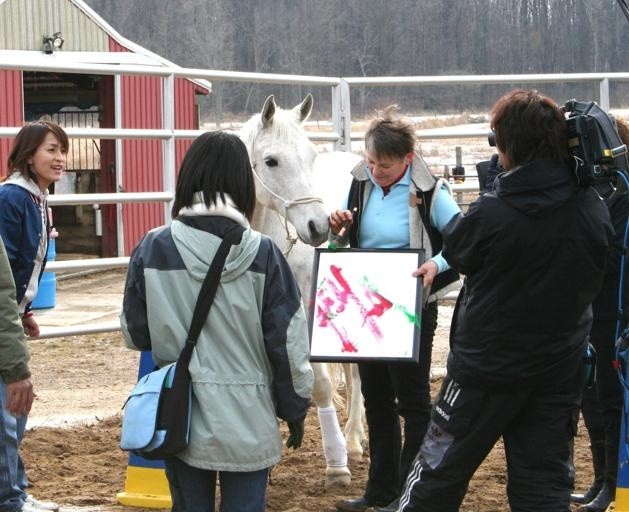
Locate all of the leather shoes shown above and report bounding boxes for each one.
[335,498,367,512]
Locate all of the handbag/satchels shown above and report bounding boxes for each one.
[122,365,194,460]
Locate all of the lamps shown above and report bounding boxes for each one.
[45,31,64,54]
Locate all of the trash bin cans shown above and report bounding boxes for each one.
[29,238,56,309]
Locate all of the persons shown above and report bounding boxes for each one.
[0,120,70,512]
[327,105,464,511]
[118,130,315,512]
[398,91,618,510]
[571,117,629,511]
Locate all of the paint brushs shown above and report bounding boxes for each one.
[328,206,358,251]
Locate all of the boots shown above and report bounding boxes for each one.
[577,440,618,512]
[570,442,601,504]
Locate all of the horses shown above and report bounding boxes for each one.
[238,92,367,489]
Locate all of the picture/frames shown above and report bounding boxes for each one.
[306,248,425,363]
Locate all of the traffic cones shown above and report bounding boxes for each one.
[115,342,174,509]
[603,366,628,512]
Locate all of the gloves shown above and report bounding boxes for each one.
[285,421,304,449]
